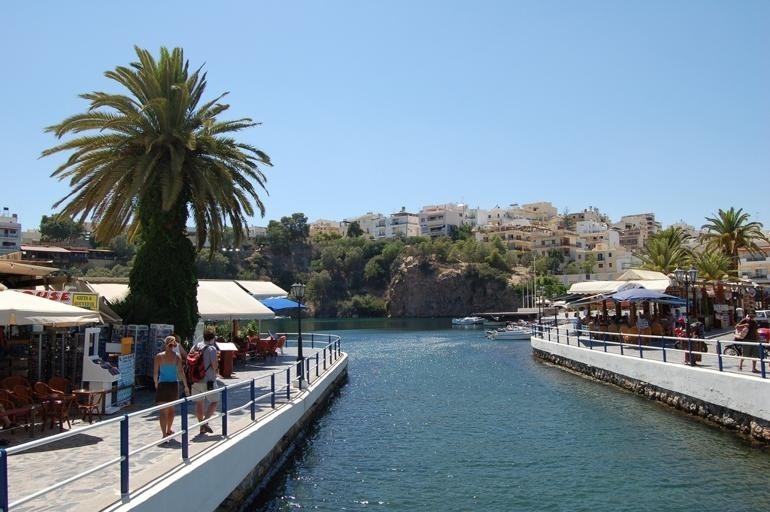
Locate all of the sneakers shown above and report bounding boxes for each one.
[202,424,213,433]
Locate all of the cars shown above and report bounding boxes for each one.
[736,308,770,345]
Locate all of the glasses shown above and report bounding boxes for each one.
[169,343,177,348]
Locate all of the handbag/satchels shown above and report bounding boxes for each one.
[735,327,750,340]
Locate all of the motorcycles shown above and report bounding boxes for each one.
[722,330,770,359]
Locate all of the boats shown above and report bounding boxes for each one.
[450,316,551,340]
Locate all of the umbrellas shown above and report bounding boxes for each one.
[0,289,105,379]
[608,288,679,318]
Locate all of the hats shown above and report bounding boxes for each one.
[748,310,757,317]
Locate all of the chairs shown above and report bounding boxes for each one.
[217,334,287,367]
[583,318,665,347]
[0,376,104,444]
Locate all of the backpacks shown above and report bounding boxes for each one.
[184,343,212,385]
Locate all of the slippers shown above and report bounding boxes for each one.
[753,369,761,373]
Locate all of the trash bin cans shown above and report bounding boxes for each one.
[685,338,702,366]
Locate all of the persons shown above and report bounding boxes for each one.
[173,331,261,405]
[152,335,191,444]
[734,309,762,373]
[190,323,220,434]
[736,306,744,323]
[573,309,706,366]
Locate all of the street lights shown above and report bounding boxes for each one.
[535,288,543,338]
[674,268,700,366]
[289,282,309,389]
